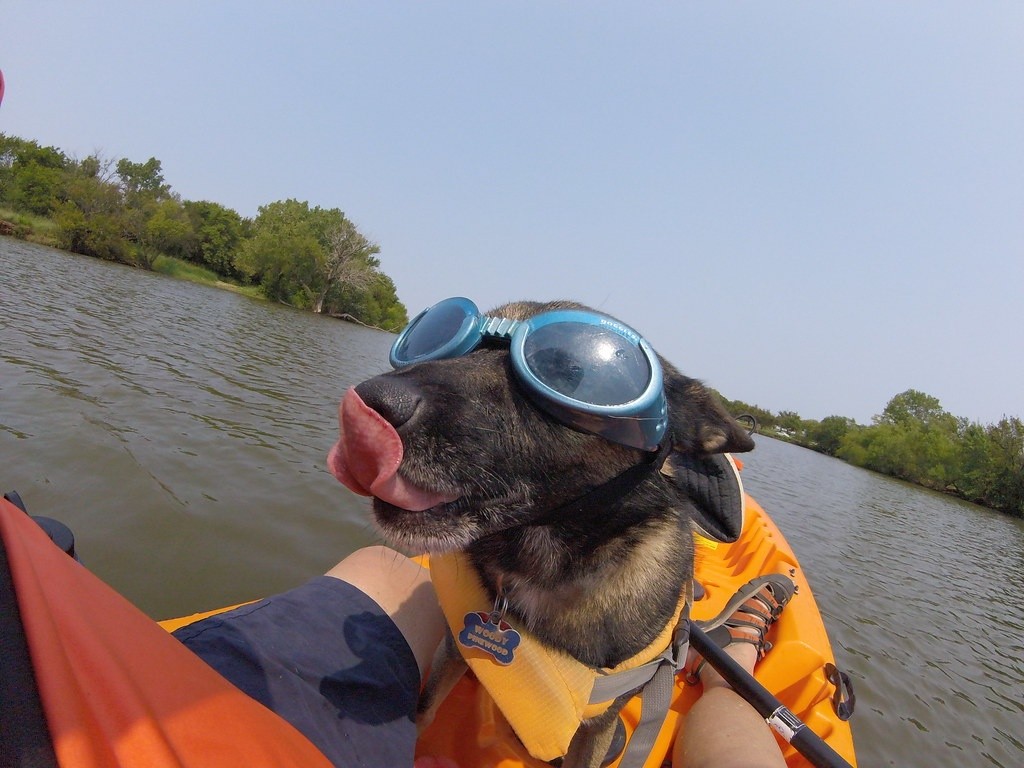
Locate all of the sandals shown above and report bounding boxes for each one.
[669,573,795,687]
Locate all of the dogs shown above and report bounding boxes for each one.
[325,296,756,768]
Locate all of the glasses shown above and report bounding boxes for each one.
[388,296,669,452]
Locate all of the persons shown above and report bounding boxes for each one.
[0,492,797,768]
